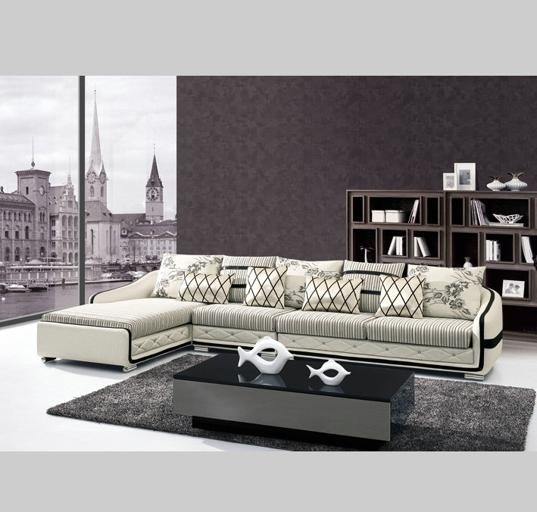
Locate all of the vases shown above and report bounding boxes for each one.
[486,171,528,192]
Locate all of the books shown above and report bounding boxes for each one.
[469,199,523,228]
[520,236,535,265]
[386,236,404,257]
[484,237,500,264]
[414,238,429,257]
[406,200,420,223]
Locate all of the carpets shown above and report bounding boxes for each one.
[46,354,535,450]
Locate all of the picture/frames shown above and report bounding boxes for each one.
[443,162,476,191]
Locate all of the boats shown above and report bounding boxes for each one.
[1,282,49,293]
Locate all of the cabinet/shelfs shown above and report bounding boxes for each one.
[344,188,536,342]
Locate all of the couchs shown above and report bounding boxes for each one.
[36,253,504,381]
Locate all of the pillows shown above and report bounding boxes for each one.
[375,273,424,319]
[152,254,219,298]
[177,272,235,305]
[302,273,361,314]
[343,258,405,312]
[275,256,343,309]
[243,265,287,310]
[221,255,275,303]
[407,263,486,321]
